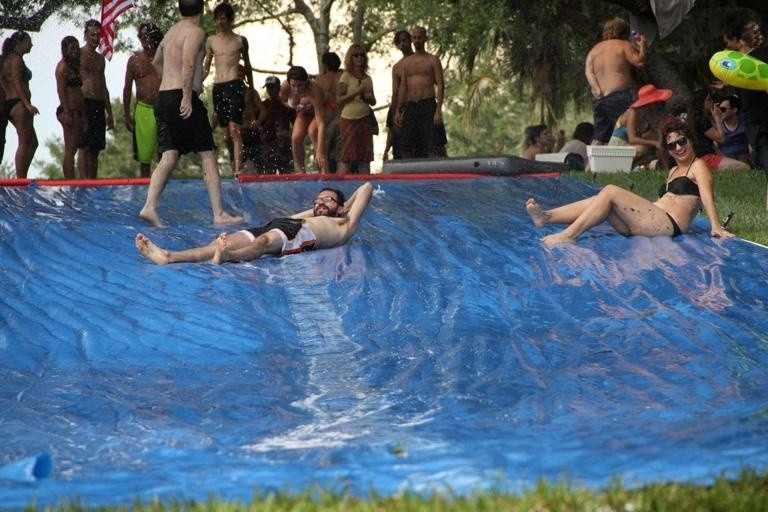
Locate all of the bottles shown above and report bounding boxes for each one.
[631,30,647,45]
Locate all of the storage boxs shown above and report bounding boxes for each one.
[533,150,569,163]
[583,143,635,176]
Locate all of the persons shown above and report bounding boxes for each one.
[520,17,768,172]
[383,24,448,160]
[523,118,735,247]
[210,44,378,175]
[134,181,373,265]
[137,0,247,230]
[1,19,166,177]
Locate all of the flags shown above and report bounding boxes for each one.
[96,1,137,62]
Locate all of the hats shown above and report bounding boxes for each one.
[629,83,674,110]
[263,76,281,89]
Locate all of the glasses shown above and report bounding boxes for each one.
[720,106,731,113]
[666,136,689,151]
[313,196,343,207]
